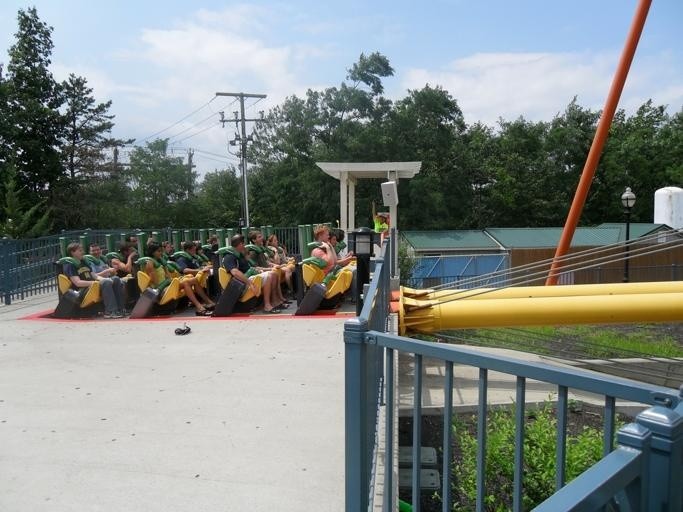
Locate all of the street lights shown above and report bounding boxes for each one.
[620,184,637,282]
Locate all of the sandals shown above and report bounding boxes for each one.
[196,304,216,316]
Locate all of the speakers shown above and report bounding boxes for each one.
[381,182,399,207]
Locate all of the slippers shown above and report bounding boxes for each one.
[263,292,297,313]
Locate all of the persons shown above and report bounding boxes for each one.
[310,224,357,306]
[371,199,389,234]
[66,230,295,319]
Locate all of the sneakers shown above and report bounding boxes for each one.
[351,301,356,305]
[104,311,131,319]
